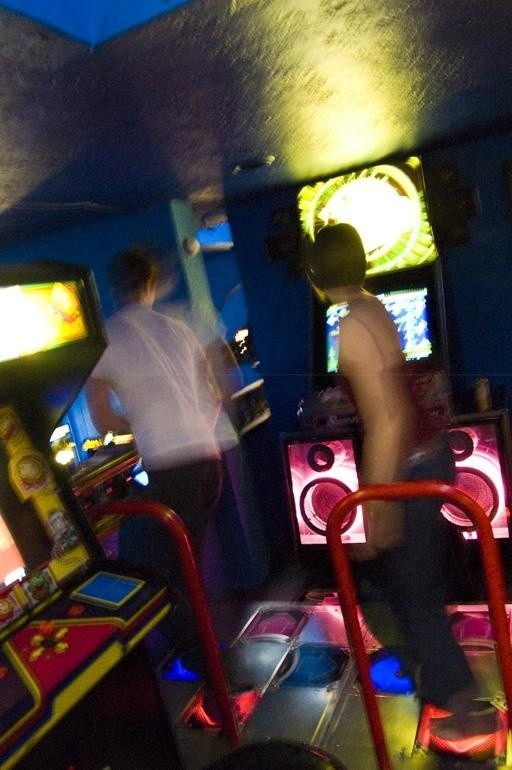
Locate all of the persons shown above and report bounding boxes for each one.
[308,221,500,739]
[88,243,241,686]
